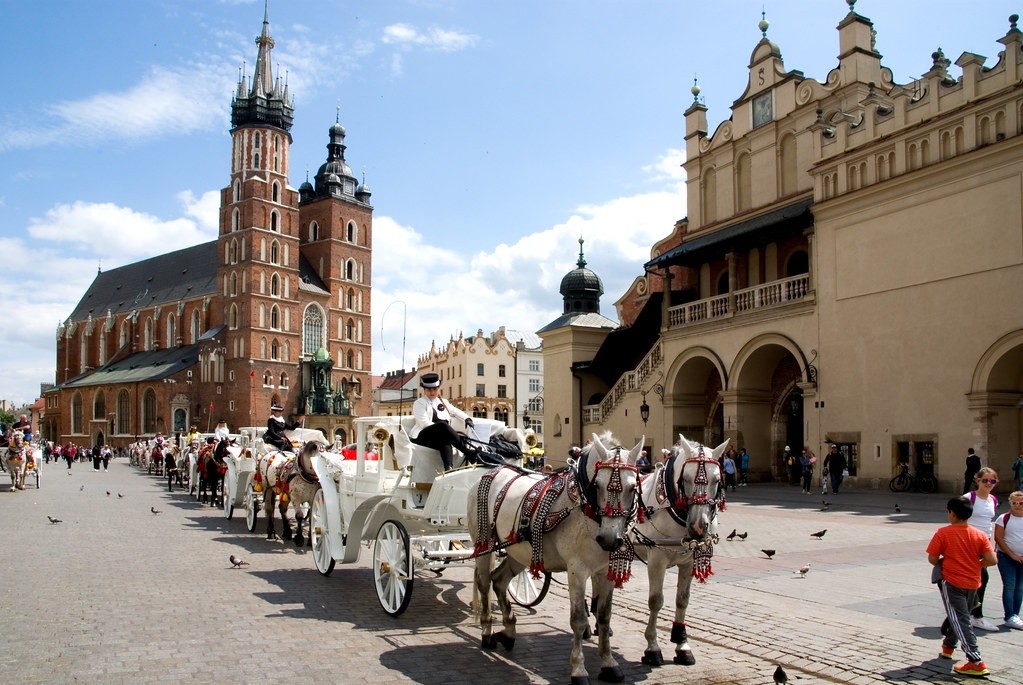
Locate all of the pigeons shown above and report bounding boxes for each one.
[117,492,124,499]
[229,555,251,569]
[772,662,788,685]
[150,506,164,516]
[894,503,901,513]
[106,490,111,496]
[810,528,828,540]
[759,549,776,560]
[47,515,63,525]
[67,471,72,476]
[726,528,748,541]
[79,484,85,491]
[820,499,833,512]
[793,562,812,578]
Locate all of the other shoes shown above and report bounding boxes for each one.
[742,483,748,486]
[971,617,1000,631]
[1004,615,1023,629]
[807,492,812,495]
[833,492,837,495]
[811,470,813,473]
[802,489,806,493]
[738,483,743,486]
[445,467,453,471]
[467,445,482,464]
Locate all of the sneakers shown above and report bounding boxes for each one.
[954,661,990,677]
[942,639,954,658]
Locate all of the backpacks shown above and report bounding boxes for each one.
[489,435,522,457]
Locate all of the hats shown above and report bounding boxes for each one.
[218,418,227,423]
[420,373,441,388]
[271,405,285,411]
[785,446,791,451]
[831,444,836,448]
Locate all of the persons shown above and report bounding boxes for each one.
[808,451,817,474]
[175,427,185,447]
[726,445,749,487]
[13,415,33,441]
[636,451,651,473]
[823,444,846,495]
[187,425,204,445]
[64,442,76,469]
[800,448,813,496]
[962,447,982,495]
[214,419,229,441]
[992,491,1023,629]
[262,404,306,451]
[783,446,800,483]
[101,445,111,472]
[958,467,1000,632]
[154,432,165,450]
[723,454,737,492]
[165,447,186,492]
[1011,455,1023,491]
[407,373,476,473]
[91,445,102,472]
[44,441,92,465]
[927,496,998,677]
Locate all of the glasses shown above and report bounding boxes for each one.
[1013,501,1023,506]
[982,479,996,484]
[802,453,805,454]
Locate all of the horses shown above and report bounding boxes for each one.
[465,425,647,684]
[0,427,30,492]
[128,431,191,493]
[195,437,237,509]
[582,432,731,668]
[256,440,378,548]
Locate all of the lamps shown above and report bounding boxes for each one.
[639,370,666,430]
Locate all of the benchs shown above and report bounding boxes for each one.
[201,432,243,448]
[385,413,530,484]
[248,426,326,454]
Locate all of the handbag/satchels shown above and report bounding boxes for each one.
[788,457,793,465]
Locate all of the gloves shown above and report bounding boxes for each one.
[465,418,475,430]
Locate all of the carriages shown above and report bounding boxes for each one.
[129,426,241,508]
[238,427,348,547]
[310,416,732,685]
[0,429,42,489]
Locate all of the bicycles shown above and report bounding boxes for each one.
[889,462,938,494]
